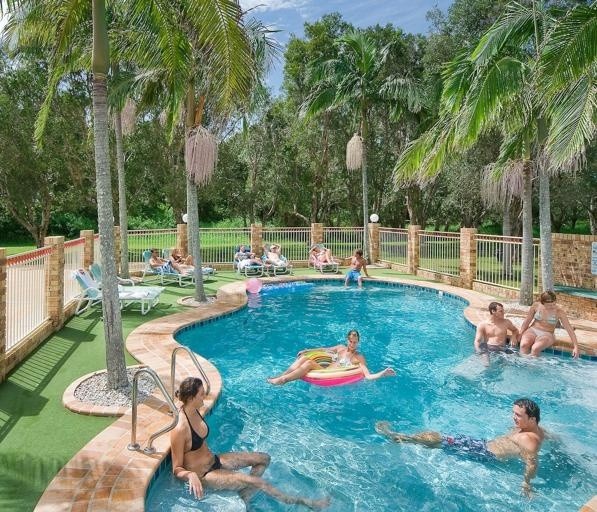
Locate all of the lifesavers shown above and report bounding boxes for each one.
[297,349,365,385]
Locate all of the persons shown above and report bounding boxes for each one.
[474,302,519,368]
[308,246,339,265]
[374,398,559,500]
[149,248,189,278]
[266,330,396,385]
[516,290,579,360]
[344,250,370,290]
[262,243,293,267]
[234,245,273,269]
[170,377,331,512]
[170,249,195,266]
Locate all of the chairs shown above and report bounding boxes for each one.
[261,244,292,277]
[90,263,165,307]
[232,245,264,278]
[72,268,151,315]
[162,248,216,284]
[308,245,341,273]
[139,250,194,287]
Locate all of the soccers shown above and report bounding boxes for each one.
[245,278,261,294]
